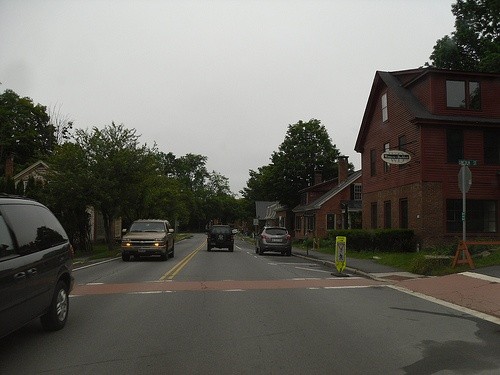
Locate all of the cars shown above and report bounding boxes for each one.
[255,226,292,256]
[232,229,241,234]
[0,194,74,340]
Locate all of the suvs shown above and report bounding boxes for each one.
[204,225,234,252]
[120,219,175,261]
